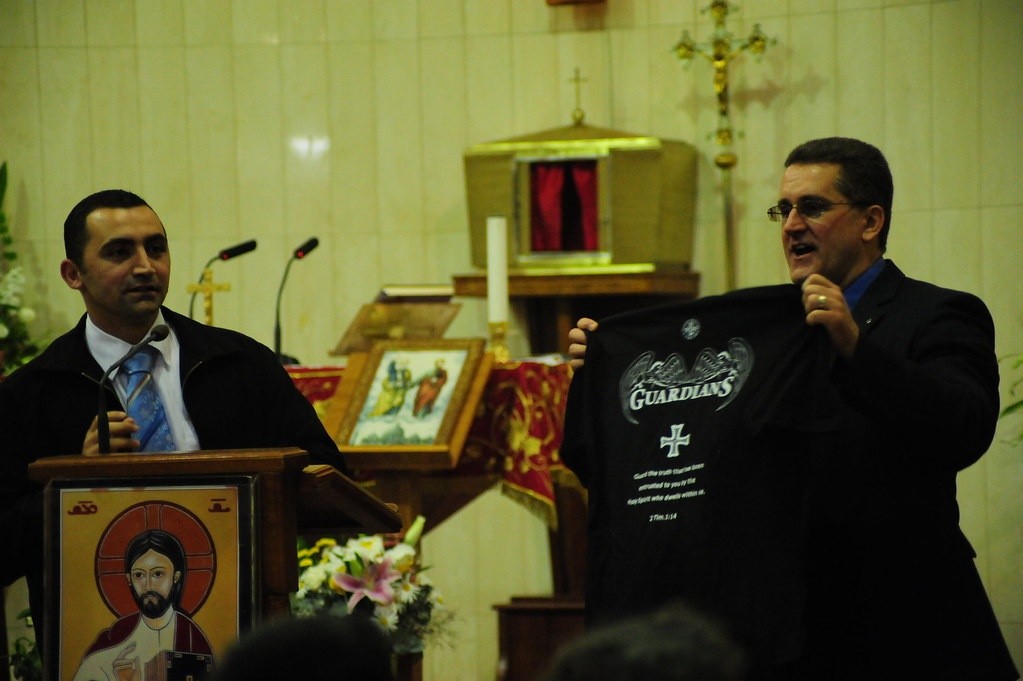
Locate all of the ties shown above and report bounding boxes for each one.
[121,345,176,450]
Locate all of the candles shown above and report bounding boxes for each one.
[485,215,507,325]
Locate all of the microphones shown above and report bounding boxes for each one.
[275,236,320,355]
[96,324,169,455]
[189,240,256,319]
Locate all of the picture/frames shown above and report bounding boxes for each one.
[517,150,613,268]
[336,335,487,446]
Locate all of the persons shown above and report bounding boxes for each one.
[568,137,1023,681]
[0,191,342,664]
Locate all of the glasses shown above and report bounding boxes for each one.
[767,201,872,222]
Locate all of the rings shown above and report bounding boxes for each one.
[819,296,826,307]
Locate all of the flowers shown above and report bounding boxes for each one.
[1,161,56,380]
[289,515,469,654]
